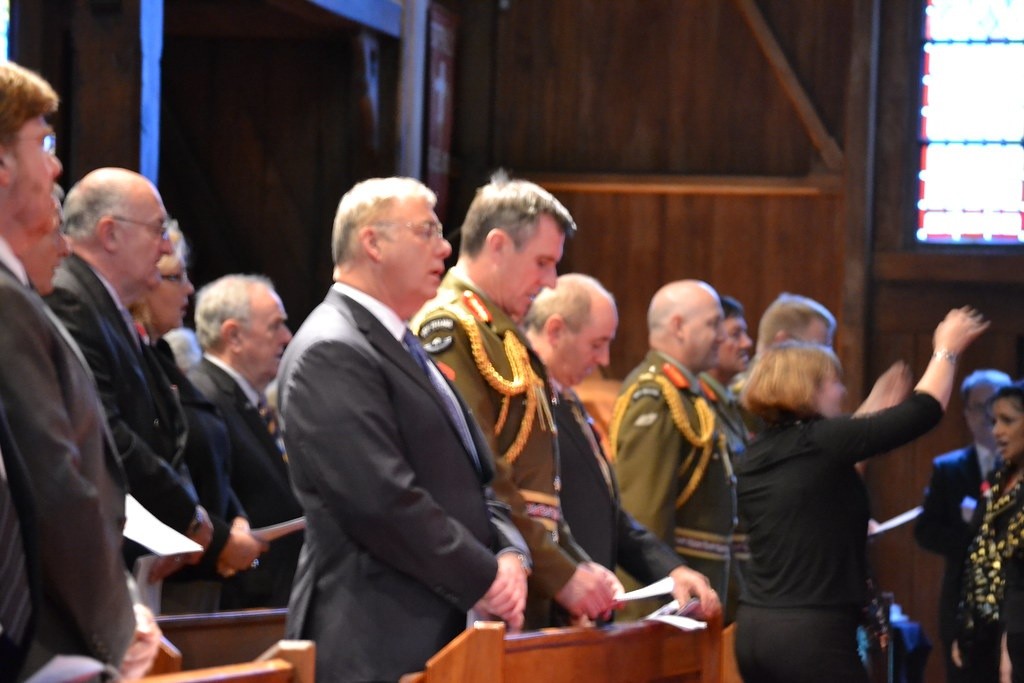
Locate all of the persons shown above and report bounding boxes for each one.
[0,59,160,683]
[57,167,1024,683]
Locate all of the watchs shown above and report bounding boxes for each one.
[514,552,533,576]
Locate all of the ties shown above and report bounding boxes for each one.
[566,394,615,500]
[404,328,480,470]
[257,395,288,463]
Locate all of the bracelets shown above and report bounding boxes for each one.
[189,510,203,532]
[935,351,960,364]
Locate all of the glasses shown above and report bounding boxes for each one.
[162,272,187,285]
[112,215,168,241]
[389,221,443,241]
[13,130,56,156]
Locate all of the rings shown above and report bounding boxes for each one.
[252,559,259,568]
